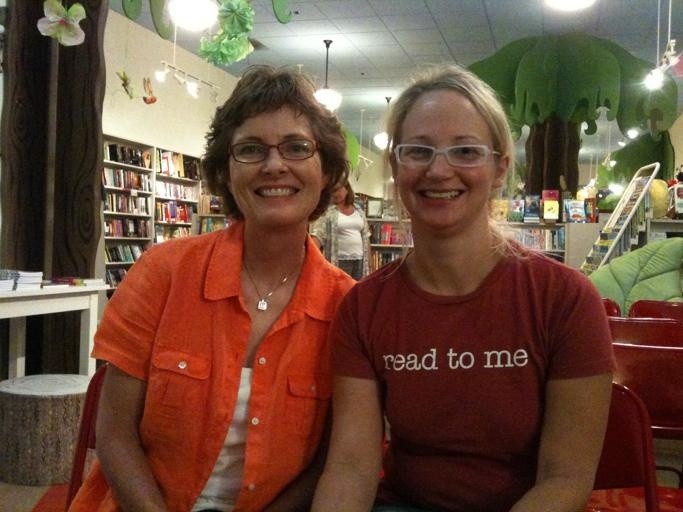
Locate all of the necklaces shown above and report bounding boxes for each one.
[241,246,308,311]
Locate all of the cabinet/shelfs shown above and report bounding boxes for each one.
[95,127,233,318]
[366,219,602,270]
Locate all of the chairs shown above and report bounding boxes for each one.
[628,299,683,319]
[65,367,107,512]
[585,382,683,512]
[612,340,682,488]
[607,315,683,346]
[602,295,620,317]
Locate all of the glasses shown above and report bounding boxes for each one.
[230,139,318,163]
[394,144,500,167]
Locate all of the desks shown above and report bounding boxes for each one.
[0,279,109,377]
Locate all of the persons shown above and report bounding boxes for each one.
[307,61,618,512]
[62,63,360,511]
[309,175,371,282]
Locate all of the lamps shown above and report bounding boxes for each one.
[154,59,203,100]
[645,0,679,89]
[167,0,220,32]
[617,136,626,147]
[627,127,637,139]
[314,39,342,111]
[373,96,394,149]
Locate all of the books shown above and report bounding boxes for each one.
[103,142,152,170]
[580,174,653,278]
[102,192,152,216]
[104,218,152,238]
[154,225,192,244]
[41,283,69,289]
[367,223,412,245]
[50,277,106,287]
[198,216,232,235]
[102,167,152,193]
[0,278,15,293]
[104,241,149,263]
[154,200,193,223]
[155,181,198,201]
[494,225,565,250]
[155,150,200,180]
[368,250,403,277]
[0,268,43,293]
[104,266,131,289]
[489,189,598,224]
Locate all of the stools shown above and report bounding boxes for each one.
[0,374,92,486]
